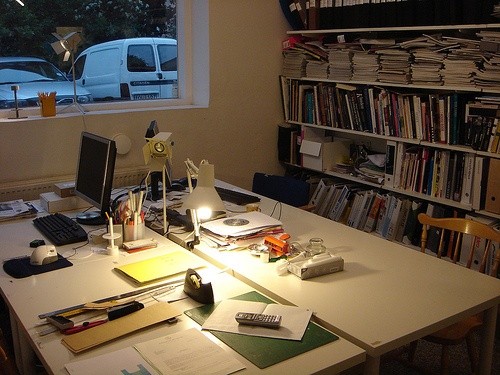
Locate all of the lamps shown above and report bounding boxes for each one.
[184,158,226,250]
[51,31,89,115]
[148,132,175,238]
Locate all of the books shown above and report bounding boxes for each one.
[278,27,500,282]
[0,202,38,221]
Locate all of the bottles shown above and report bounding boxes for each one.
[306,238,326,255]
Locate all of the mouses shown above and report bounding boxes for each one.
[171,177,194,190]
[30,243,58,266]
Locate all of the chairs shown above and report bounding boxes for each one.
[388,213,500,374]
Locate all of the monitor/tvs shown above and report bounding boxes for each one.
[72,132,115,226]
[145,120,173,201]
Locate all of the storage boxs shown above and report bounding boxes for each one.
[299,126,351,173]
[39,192,92,212]
[54,182,76,198]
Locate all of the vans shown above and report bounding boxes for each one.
[66,37,178,103]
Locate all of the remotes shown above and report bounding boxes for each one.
[235,312,282,327]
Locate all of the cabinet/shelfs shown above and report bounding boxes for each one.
[284,23,500,272]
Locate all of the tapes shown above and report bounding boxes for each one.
[190,275,201,288]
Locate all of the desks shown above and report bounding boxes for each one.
[0,177,500,375]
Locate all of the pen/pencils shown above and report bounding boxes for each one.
[108,206,145,249]
[128,198,130,209]
[132,194,136,211]
[130,191,132,211]
[105,212,110,220]
[137,190,144,217]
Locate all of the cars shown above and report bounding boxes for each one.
[0,57,95,109]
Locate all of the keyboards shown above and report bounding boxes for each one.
[214,186,262,206]
[33,213,88,246]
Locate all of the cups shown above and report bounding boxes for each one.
[125,221,145,242]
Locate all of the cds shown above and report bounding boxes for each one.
[223,218,250,226]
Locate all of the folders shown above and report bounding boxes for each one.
[113,250,208,287]
